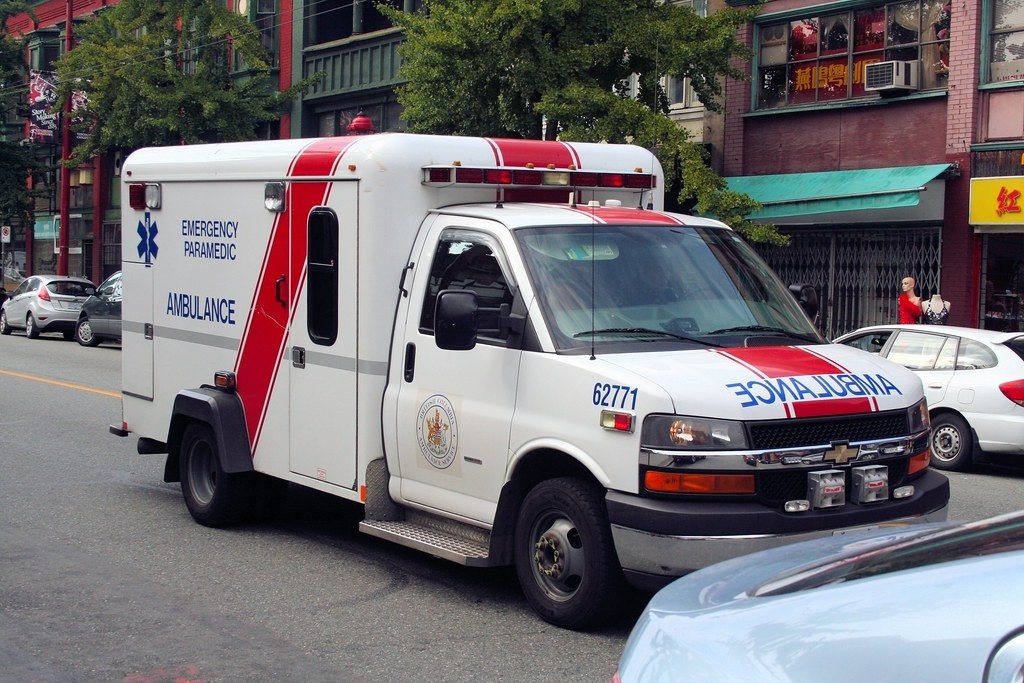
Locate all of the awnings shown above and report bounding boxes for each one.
[689,164,955,222]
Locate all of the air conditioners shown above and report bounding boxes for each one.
[864,60,918,92]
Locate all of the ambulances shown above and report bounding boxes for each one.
[109,109,951,630]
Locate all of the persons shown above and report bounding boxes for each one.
[896,277,923,325]
[635,251,678,303]
[924,291,951,325]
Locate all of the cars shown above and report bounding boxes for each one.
[74,270,122,346]
[830,324,1024,470]
[0,275,98,340]
[609,508,1024,683]
